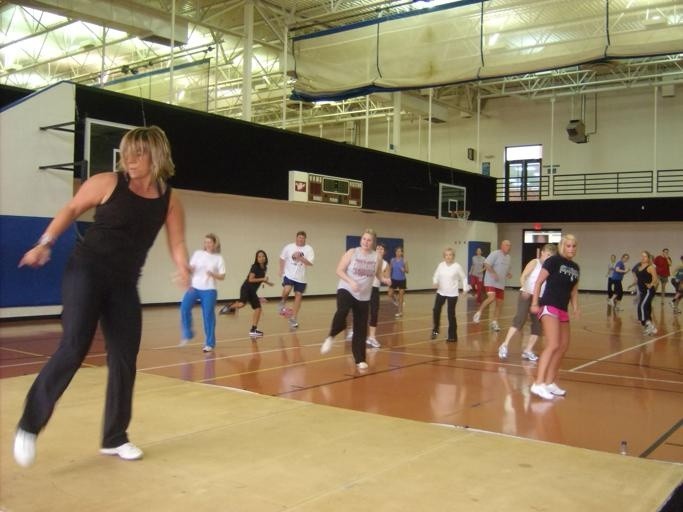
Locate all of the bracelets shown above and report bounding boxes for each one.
[38,232,56,246]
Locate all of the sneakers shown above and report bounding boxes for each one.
[13,428,37,467]
[608,297,681,337]
[100,442,143,460]
[521,350,538,360]
[498,344,509,359]
[531,382,554,400]
[548,381,566,396]
[393,294,500,341]
[319,329,381,368]
[177,303,298,352]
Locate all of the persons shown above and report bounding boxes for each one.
[469,239,559,361]
[176,233,225,353]
[430,248,469,344]
[277,231,314,327]
[529,234,582,400]
[218,249,275,336]
[12,125,191,470]
[319,227,409,369]
[605,249,683,334]
